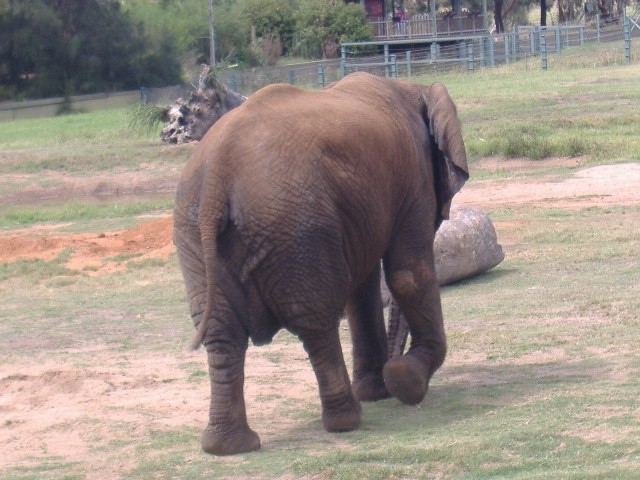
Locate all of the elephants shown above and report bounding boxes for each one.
[173,71,470,455]
